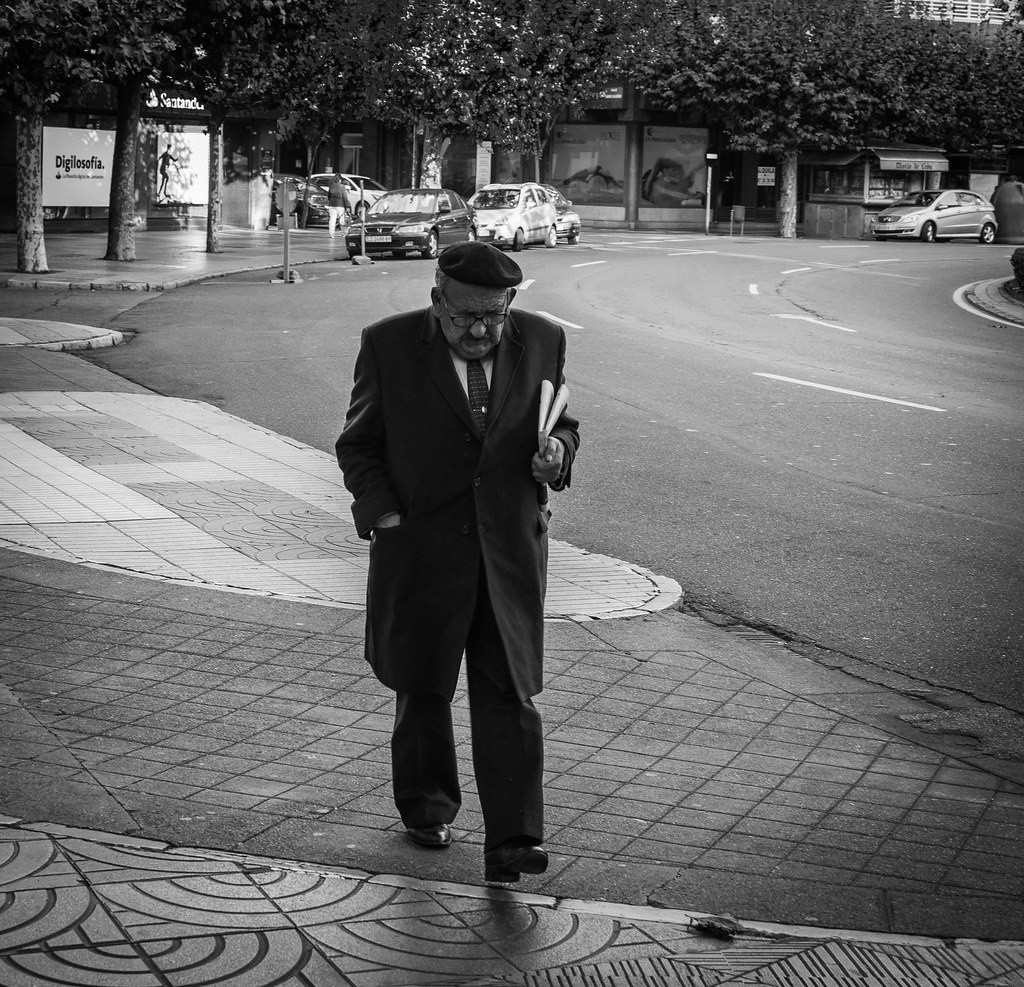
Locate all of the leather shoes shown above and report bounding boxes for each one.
[409,823,452,845]
[485,840,548,883]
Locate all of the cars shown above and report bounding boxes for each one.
[346,188,476,258]
[538,182,582,245]
[272,173,350,226]
[869,189,999,244]
[305,172,387,217]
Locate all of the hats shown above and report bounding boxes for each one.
[438,241,523,287]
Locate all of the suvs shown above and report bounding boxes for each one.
[468,181,557,251]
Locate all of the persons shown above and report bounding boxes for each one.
[328,173,351,238]
[335,243,582,882]
[558,164,622,189]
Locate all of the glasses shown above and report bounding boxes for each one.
[440,288,509,327]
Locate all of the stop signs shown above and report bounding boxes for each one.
[705,146,719,166]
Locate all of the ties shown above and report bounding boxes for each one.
[466,359,489,439]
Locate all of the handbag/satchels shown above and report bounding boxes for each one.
[339,211,352,226]
